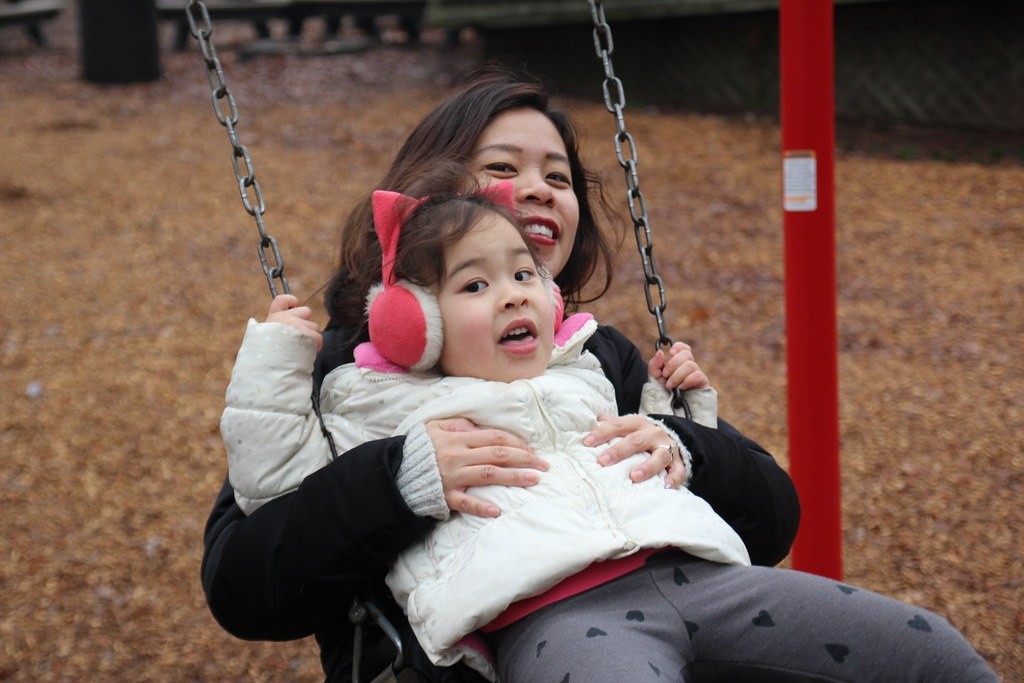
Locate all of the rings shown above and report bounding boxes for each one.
[660,443,675,463]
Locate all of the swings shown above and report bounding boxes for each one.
[183,1,687,682]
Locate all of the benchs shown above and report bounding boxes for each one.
[157,0,424,53]
[0,0,59,48]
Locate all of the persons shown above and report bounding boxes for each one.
[218,157,1000,683]
[200,74,826,683]
